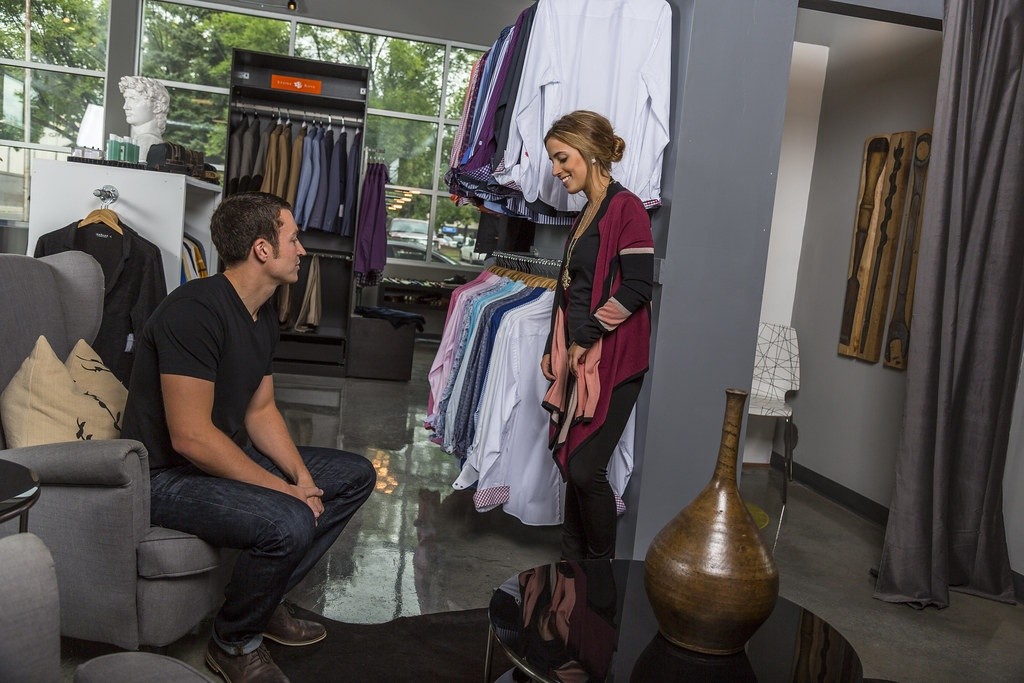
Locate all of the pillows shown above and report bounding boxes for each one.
[0,335,129,448]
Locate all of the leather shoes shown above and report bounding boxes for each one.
[261,606,327,646]
[206,635,290,683]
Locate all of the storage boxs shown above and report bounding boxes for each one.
[270,74,323,95]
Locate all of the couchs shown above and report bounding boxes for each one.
[0,530,217,683]
[0,249,239,652]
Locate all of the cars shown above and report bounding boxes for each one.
[386,242,461,266]
[436,233,473,249]
[460,238,488,264]
[390,218,441,254]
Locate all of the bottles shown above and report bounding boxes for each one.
[641,387,780,657]
[107,133,140,163]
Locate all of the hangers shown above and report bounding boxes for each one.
[301,111,307,128]
[312,112,316,129]
[319,113,323,129]
[325,113,332,131]
[78,189,123,235]
[253,103,259,119]
[355,116,360,134]
[485,251,562,291]
[285,108,292,124]
[339,115,346,133]
[241,103,248,114]
[272,106,275,119]
[276,107,282,125]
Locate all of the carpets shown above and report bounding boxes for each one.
[260,603,516,683]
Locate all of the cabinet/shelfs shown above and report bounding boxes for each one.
[223,47,370,379]
[24,158,222,295]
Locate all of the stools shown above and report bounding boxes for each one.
[346,313,415,381]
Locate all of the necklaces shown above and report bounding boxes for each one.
[560,179,617,290]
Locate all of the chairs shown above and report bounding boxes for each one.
[747,321,800,505]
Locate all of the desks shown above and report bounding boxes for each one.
[0,459,41,534]
[482,560,863,683]
[376,277,455,344]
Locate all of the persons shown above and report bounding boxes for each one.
[118,76,170,163]
[539,110,654,563]
[563,562,617,683]
[121,192,376,683]
